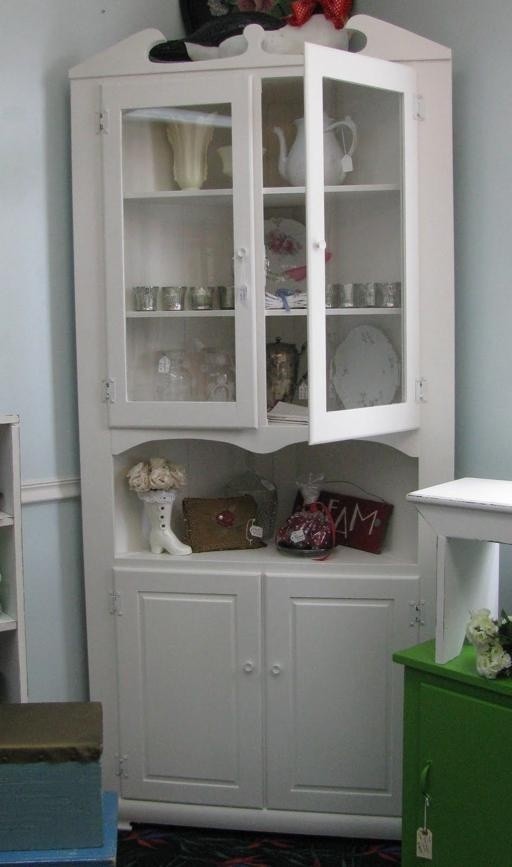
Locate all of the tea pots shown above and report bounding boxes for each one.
[268,337,308,408]
[272,109,358,186]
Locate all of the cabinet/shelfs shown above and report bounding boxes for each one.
[393,477,511,865]
[1,412,29,705]
[70,17,456,839]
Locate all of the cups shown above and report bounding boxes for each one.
[325,282,400,311]
[133,284,233,310]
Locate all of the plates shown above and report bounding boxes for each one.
[331,325,400,411]
[265,216,307,295]
